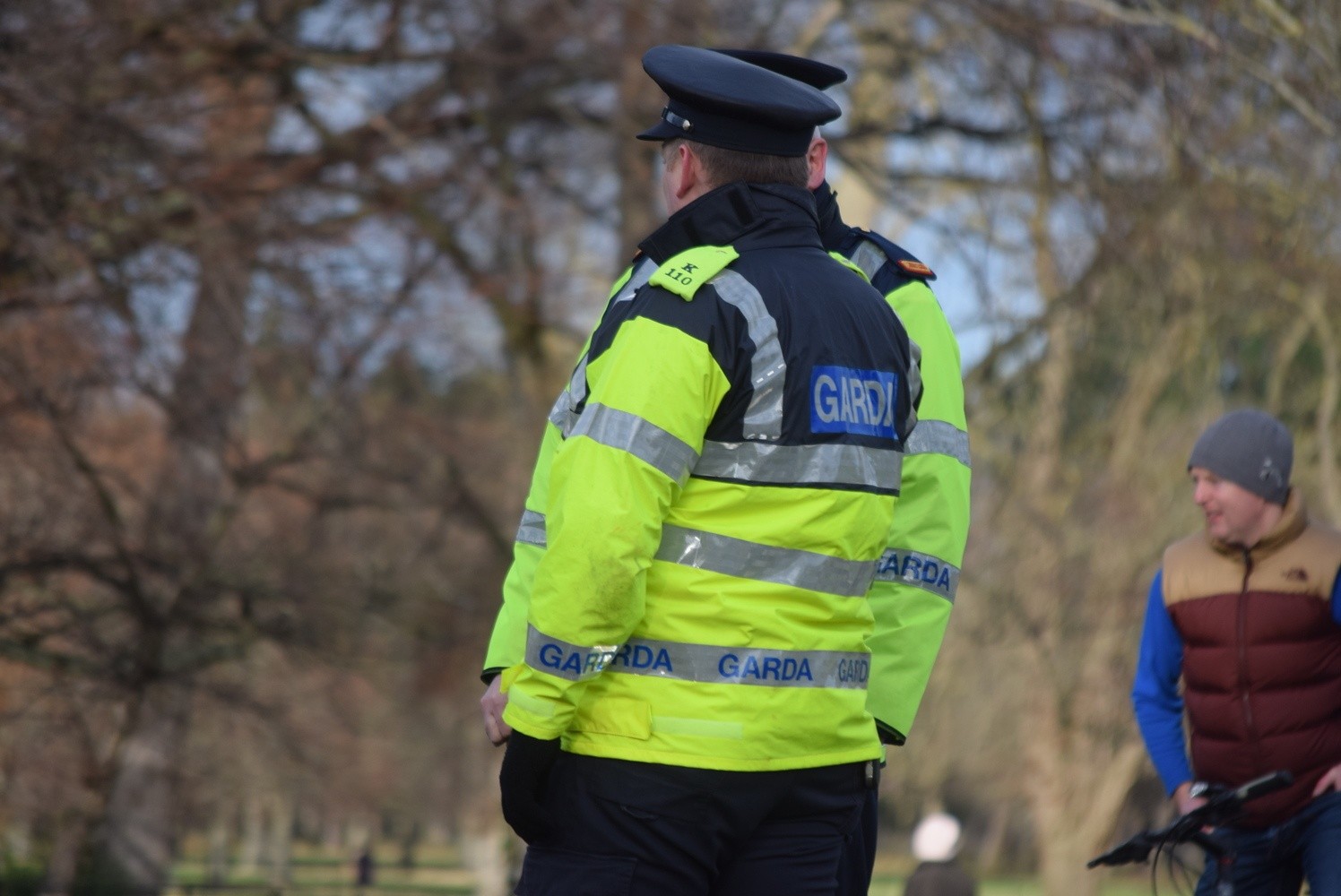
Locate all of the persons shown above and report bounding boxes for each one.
[896,812,980,896]
[356,842,377,887]
[476,42,971,895]
[1132,406,1341,893]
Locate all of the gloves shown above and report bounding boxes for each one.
[500,728,563,849]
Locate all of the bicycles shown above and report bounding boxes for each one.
[1085,771,1297,896]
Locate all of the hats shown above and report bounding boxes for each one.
[1186,408,1293,510]
[635,44,841,157]
[708,48,847,90]
